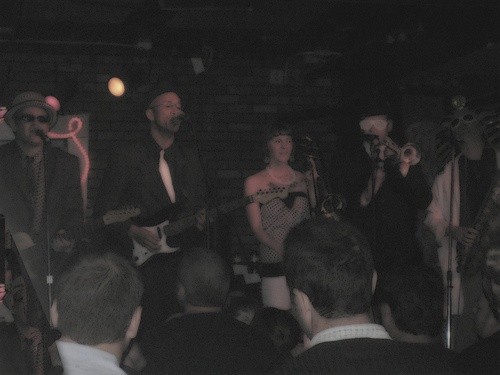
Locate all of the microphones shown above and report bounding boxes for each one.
[360,134,379,141]
[35,128,50,144]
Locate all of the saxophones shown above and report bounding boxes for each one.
[459,121,500,272]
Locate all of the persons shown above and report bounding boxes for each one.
[0,87,217,375]
[139,246,278,375]
[244,100,500,375]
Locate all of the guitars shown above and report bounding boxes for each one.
[116,186,287,272]
[38,207,143,250]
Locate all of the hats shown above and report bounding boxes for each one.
[3,91,57,132]
[145,80,183,108]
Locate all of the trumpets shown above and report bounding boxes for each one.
[376,134,422,166]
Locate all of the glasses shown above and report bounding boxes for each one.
[359,119,386,131]
[451,112,476,130]
[17,114,50,123]
[153,101,183,110]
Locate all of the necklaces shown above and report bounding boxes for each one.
[265,165,296,185]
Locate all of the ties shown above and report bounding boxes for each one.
[158,149,176,203]
